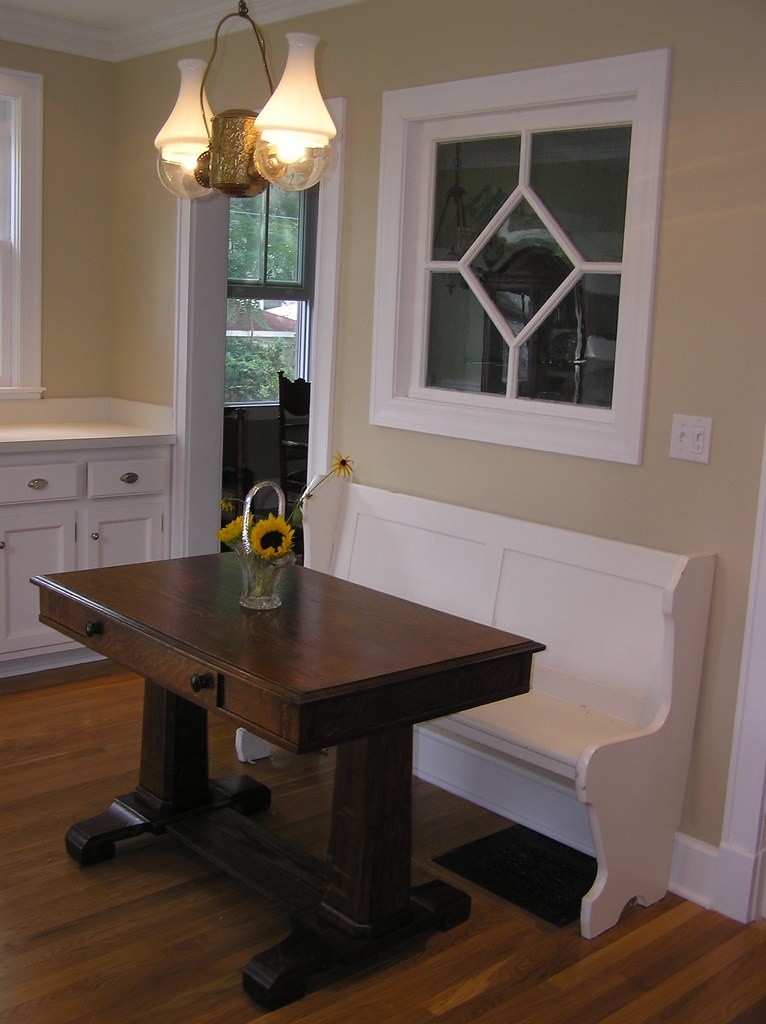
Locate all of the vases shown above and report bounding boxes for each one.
[234,545,298,609]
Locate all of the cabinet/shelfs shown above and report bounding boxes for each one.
[0,444,173,695]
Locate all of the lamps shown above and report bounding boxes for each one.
[154,0,336,199]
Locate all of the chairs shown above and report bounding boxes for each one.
[224,408,255,519]
[278,370,310,508]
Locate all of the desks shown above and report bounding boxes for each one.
[29,553,546,1010]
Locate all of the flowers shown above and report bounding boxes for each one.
[216,451,353,597]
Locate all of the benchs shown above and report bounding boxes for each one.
[236,476,718,939]
